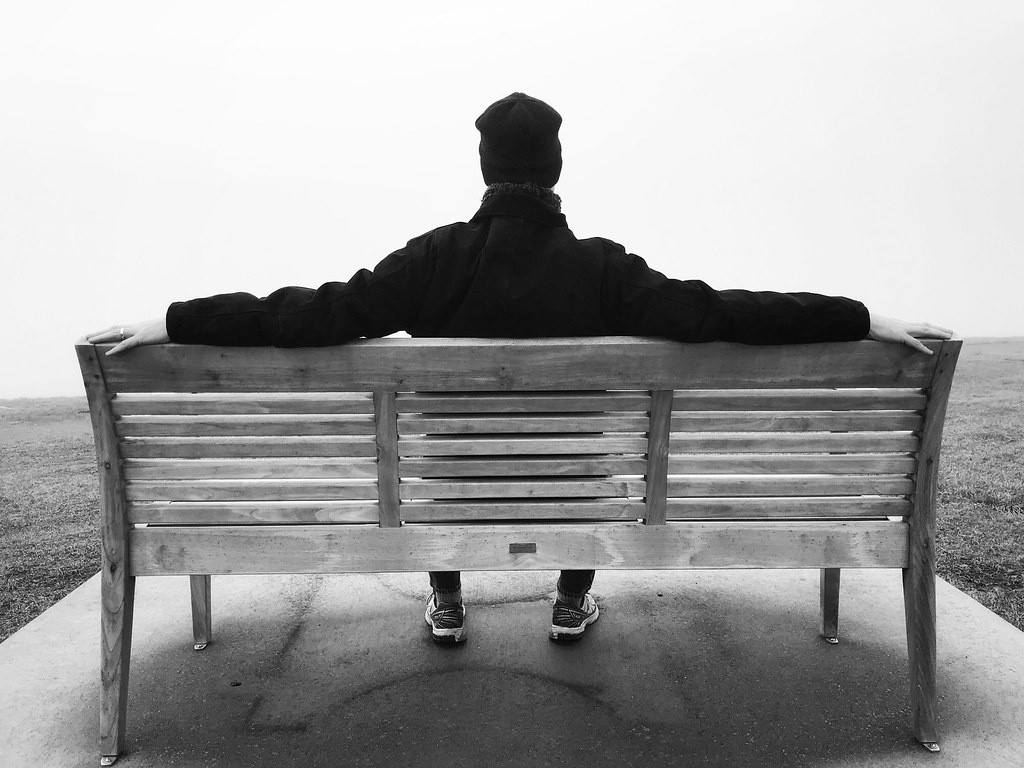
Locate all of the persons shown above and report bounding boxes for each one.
[85,91,954,645]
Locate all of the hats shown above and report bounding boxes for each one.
[476,91,562,188]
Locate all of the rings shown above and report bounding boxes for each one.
[120,328,126,340]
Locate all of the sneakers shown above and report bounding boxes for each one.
[425,591,467,644]
[548,593,599,641]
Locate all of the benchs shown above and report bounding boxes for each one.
[77,334,963,766]
